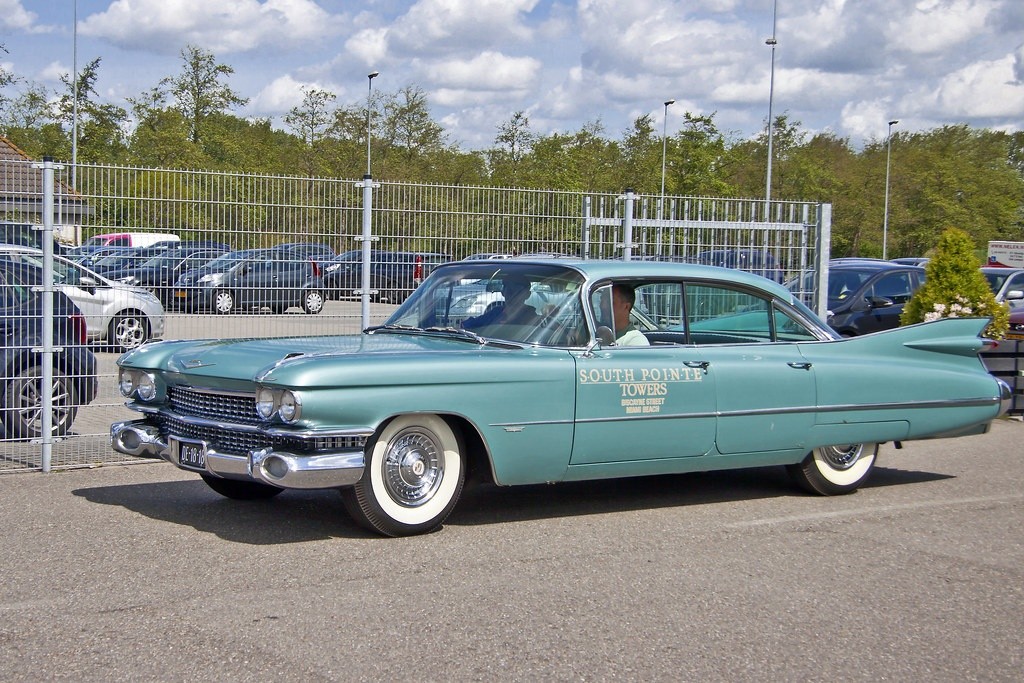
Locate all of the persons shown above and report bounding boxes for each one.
[458,272,652,348]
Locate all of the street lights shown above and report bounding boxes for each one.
[763,39,778,283]
[882,120,900,258]
[659,100,676,260]
[362,71,380,334]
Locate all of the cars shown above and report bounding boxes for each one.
[0,260,98,439]
[0,243,166,354]
[169,249,329,314]
[682,249,785,285]
[744,260,932,339]
[2,222,325,319]
[109,258,1012,539]
[976,268,1024,332]
[462,248,935,340]
[273,244,336,272]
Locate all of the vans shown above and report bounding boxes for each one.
[316,251,454,305]
